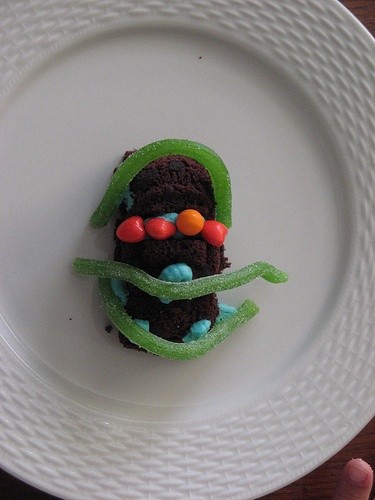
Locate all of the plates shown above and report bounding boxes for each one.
[0,0,375,500]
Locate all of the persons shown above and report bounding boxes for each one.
[335,458,373,500]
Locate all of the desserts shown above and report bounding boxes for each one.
[72,140,287,358]
